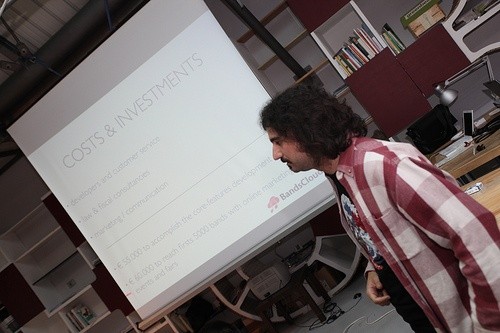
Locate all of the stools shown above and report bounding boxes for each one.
[253,263,331,332]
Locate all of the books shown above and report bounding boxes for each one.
[325,23,407,77]
[65,301,95,331]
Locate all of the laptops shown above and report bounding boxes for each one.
[439,110,474,157]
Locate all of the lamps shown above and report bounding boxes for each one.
[433,56,500,109]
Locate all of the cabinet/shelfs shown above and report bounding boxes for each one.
[0,0,500,333]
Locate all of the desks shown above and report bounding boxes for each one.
[427,107,500,188]
[460,167,499,235]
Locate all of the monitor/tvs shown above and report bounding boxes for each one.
[482,80,500,98]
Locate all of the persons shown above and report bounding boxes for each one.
[259,84,500,333]
